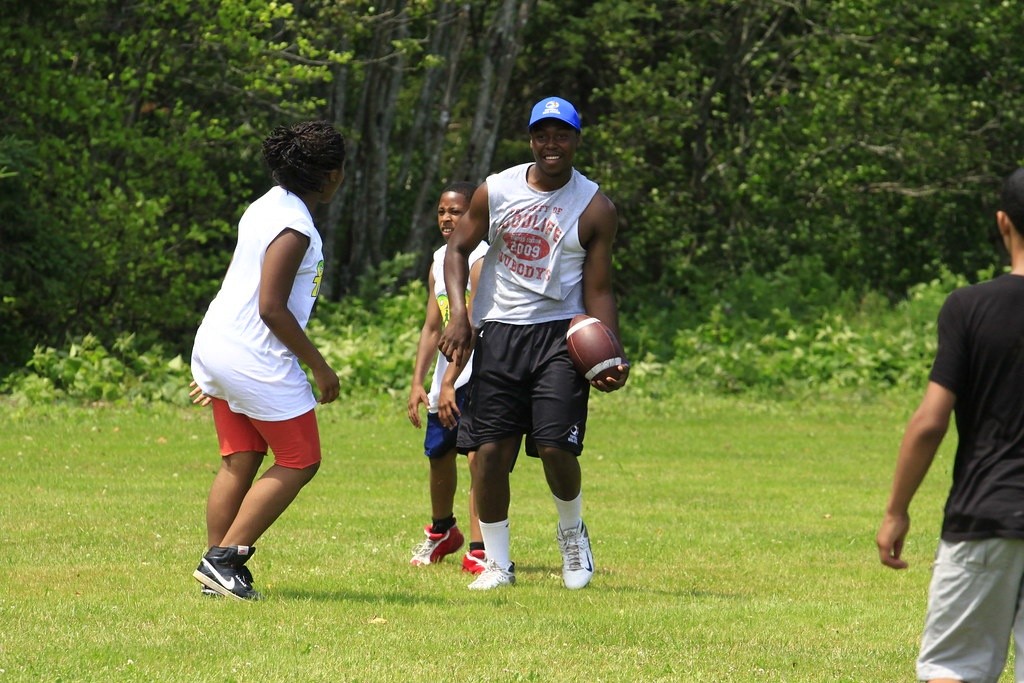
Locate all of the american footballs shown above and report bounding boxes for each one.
[566,314,622,389]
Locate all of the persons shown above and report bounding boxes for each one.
[405,183,496,575]
[188,116,348,600]
[436,98,632,590]
[876,164,1024,683]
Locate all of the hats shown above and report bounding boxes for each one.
[530,97,581,133]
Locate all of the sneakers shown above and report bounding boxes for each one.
[192,546,265,604]
[461,550,487,575]
[201,566,254,596]
[467,561,517,591]
[410,518,464,566]
[556,517,594,590]
